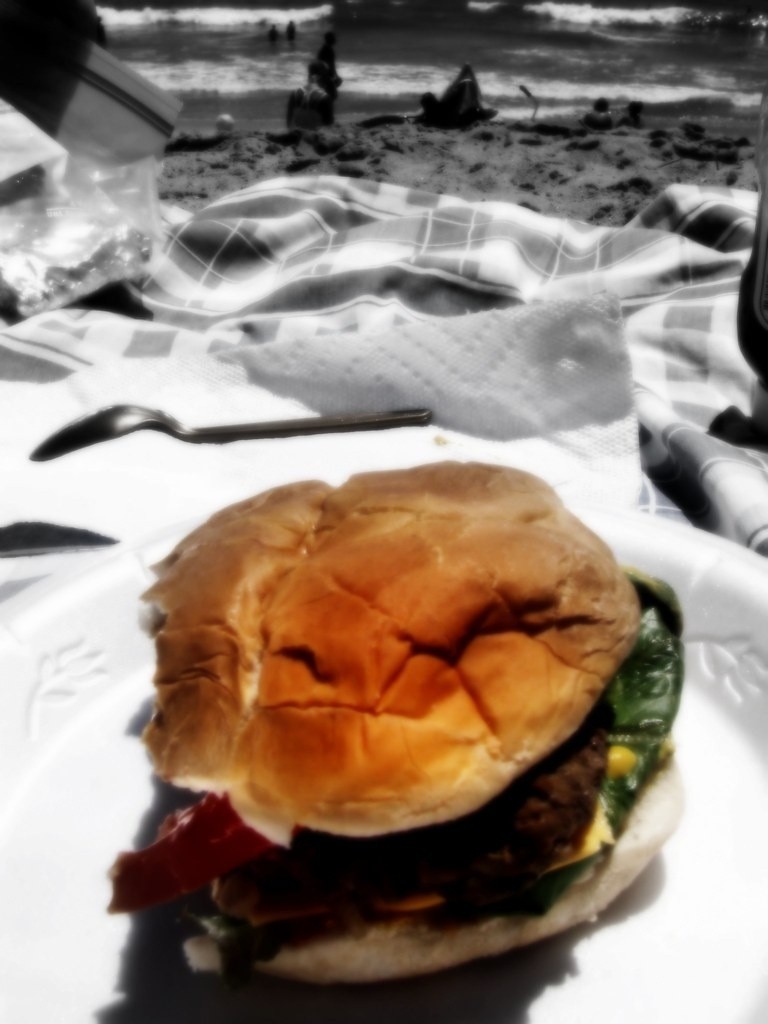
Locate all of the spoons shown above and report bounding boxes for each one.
[29,403,433,463]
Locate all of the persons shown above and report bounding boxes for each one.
[582,98,644,130]
[269,21,341,129]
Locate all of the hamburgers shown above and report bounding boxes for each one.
[101,461,689,989]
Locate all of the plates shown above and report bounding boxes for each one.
[1,487,768,1024]
